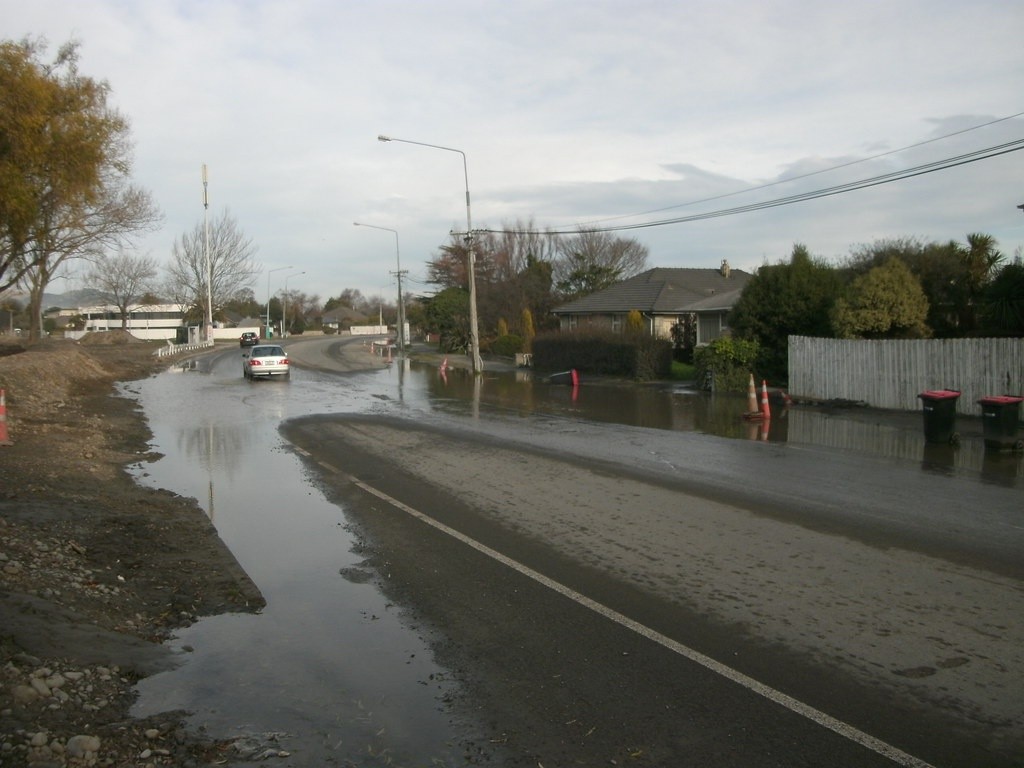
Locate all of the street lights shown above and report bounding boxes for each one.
[353,222,406,355]
[266,266,292,338]
[378,135,484,377]
[379,283,394,334]
[282,271,306,338]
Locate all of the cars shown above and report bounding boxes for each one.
[242,344,290,381]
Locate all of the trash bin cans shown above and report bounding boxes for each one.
[917,388,961,444]
[977,395,1024,451]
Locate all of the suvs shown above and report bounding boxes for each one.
[239,332,260,348]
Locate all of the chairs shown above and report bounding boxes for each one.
[271,348,277,356]
[255,350,262,356]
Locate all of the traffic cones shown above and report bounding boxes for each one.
[0,389,14,446]
[370,342,374,354]
[542,369,578,385]
[759,380,770,420]
[438,357,448,371]
[386,346,392,363]
[779,390,794,406]
[741,374,765,418]
